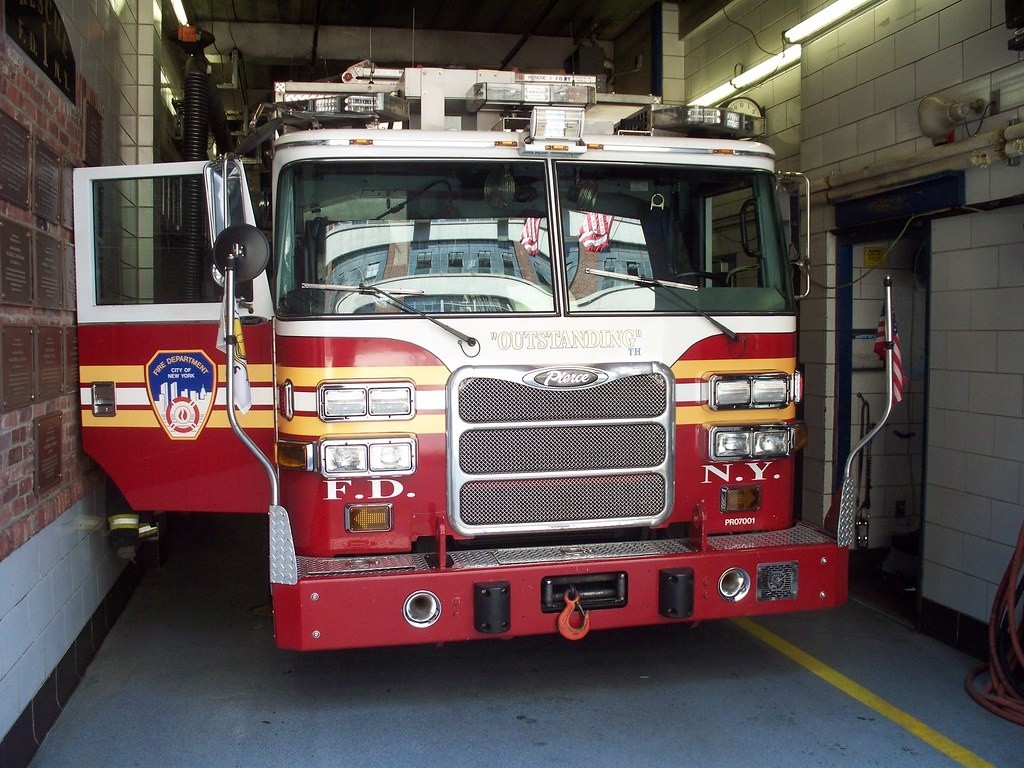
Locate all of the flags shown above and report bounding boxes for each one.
[577,213,613,252]
[874,292,907,405]
[214,275,252,413]
[520,216,542,255]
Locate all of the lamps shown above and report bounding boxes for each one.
[687,44,801,108]
[917,95,969,138]
[782,0,877,45]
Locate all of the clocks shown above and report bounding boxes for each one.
[717,97,762,141]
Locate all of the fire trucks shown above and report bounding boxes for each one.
[73,58,895,654]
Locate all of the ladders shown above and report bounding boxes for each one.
[341,64,597,90]
